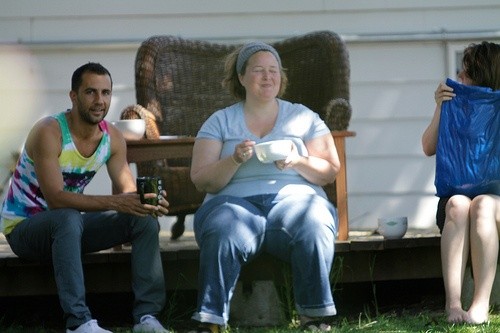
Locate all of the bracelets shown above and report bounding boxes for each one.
[230,151,242,167]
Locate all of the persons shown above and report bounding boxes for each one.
[0,61,178,333]
[422,41,500,327]
[189,40,341,333]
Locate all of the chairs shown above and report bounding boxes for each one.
[134,29,352,241]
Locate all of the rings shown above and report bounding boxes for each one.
[242,152,247,158]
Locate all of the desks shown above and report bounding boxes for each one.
[115,130,356,251]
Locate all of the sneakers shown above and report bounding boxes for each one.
[66,319,112,333]
[130,314,171,333]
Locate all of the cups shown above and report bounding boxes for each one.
[134,175,162,207]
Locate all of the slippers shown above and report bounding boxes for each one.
[188,323,220,333]
[300,318,332,333]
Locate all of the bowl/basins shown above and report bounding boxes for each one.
[377,217,408,239]
[254,138,292,164]
[111,119,146,141]
